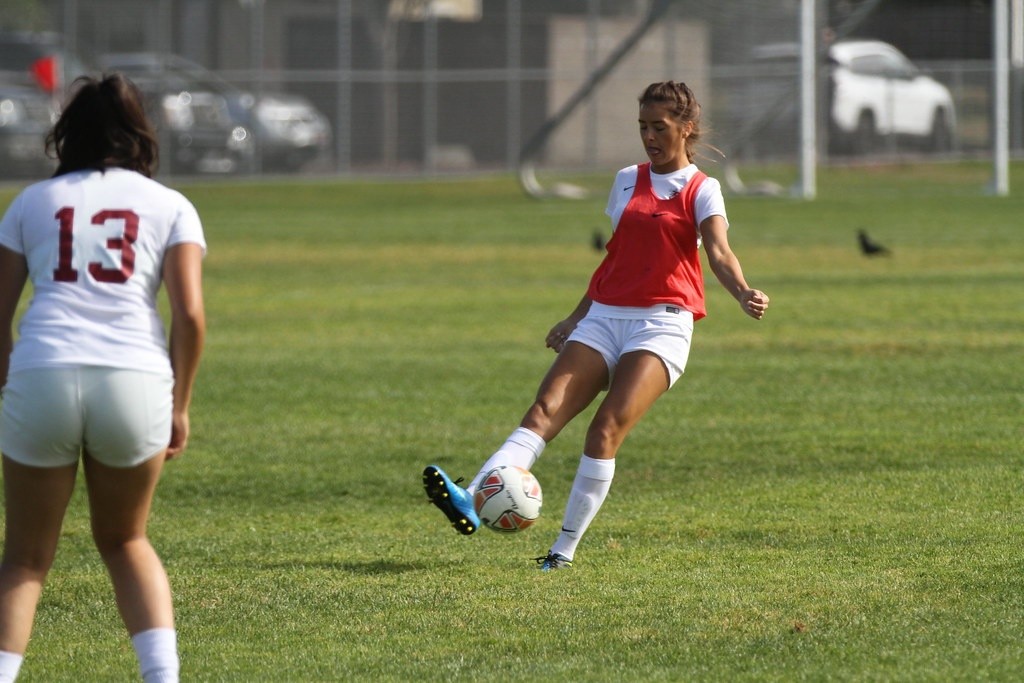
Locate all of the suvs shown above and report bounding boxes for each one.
[1,30,78,178]
[748,39,956,159]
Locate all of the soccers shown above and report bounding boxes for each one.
[473,465,544,534]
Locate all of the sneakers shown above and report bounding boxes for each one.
[529,550,573,570]
[421,465,481,535]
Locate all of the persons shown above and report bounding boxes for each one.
[420,79,771,573]
[0,69,208,683]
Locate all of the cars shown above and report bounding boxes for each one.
[78,54,330,181]
